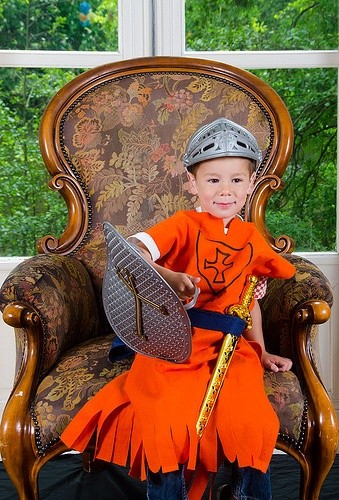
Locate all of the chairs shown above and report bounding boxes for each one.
[0,56,339,500]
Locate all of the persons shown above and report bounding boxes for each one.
[58,117,294,500]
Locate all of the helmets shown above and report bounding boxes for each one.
[182,118,263,168]
